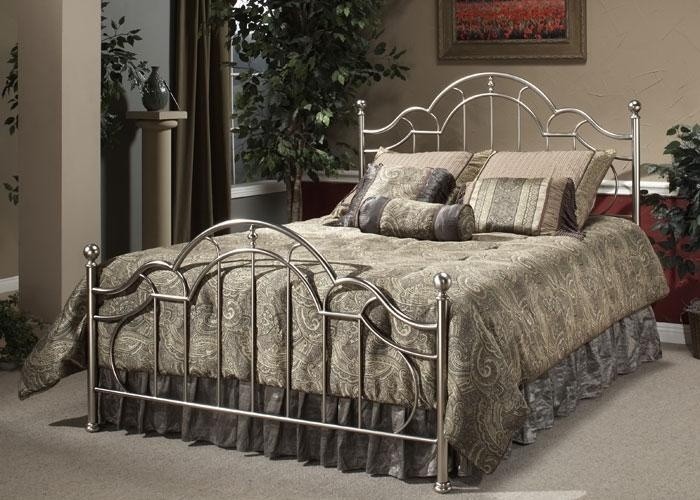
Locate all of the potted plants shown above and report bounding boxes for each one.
[639,123,699,359]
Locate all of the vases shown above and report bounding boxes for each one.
[143,67,168,111]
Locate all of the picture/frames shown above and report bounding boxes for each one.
[436,0,587,65]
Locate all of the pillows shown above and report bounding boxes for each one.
[322,145,616,240]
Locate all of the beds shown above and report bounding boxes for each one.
[84,71,641,494]
